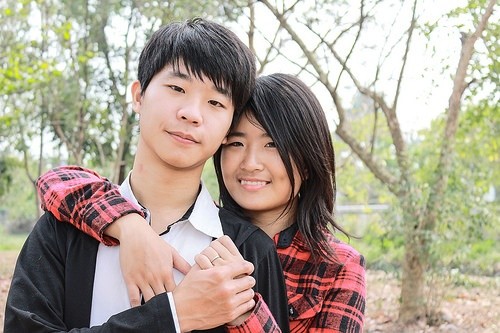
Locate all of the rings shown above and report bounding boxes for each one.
[209,256,221,264]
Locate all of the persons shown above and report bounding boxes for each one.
[2,17,292,333]
[36,71,367,333]
